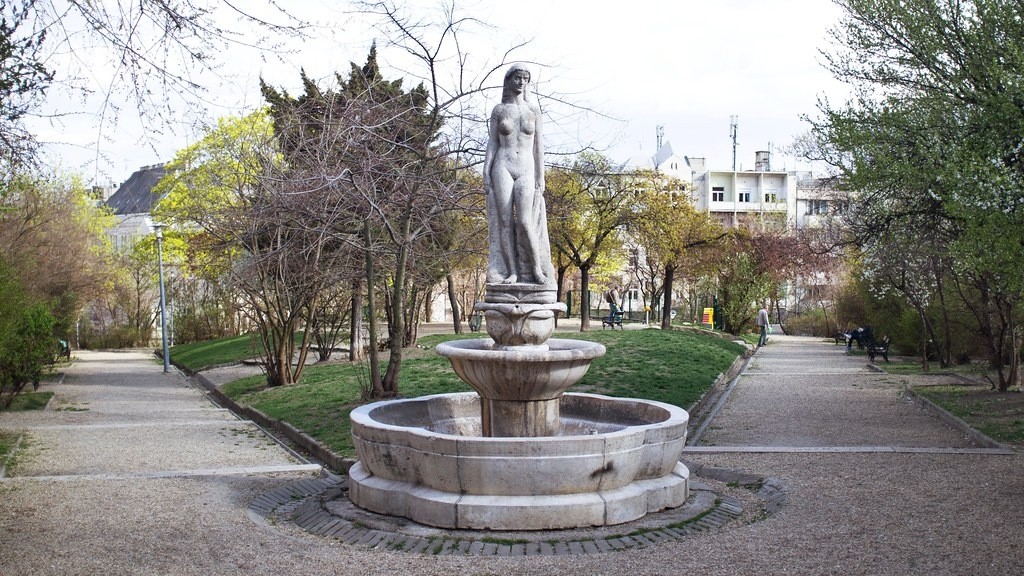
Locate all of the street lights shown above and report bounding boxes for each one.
[148,221,172,373]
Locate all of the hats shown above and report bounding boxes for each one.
[615,285,623,289]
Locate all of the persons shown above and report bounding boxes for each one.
[604,285,623,327]
[758,304,769,347]
[483,63,549,284]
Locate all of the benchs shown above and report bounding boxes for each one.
[867,335,892,362]
[57,340,71,360]
[602,311,626,331]
[834,329,850,344]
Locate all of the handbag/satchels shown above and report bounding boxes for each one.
[607,290,614,303]
